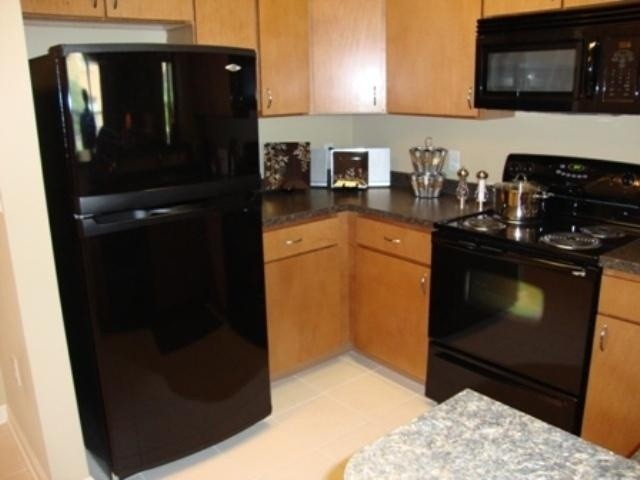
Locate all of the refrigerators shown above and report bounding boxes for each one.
[29,43,272,480]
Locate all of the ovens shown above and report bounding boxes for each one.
[425,228,603,437]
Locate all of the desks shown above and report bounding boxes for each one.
[325,386,640,479]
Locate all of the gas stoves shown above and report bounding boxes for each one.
[435,202,640,271]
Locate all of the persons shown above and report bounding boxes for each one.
[79,89,96,149]
[91,101,141,192]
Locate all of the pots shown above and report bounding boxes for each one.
[492,172,553,223]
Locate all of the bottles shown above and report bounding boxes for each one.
[455,166,470,199]
[473,169,491,200]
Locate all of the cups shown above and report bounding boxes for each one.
[410,138,446,199]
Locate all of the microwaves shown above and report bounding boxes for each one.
[472,3,640,117]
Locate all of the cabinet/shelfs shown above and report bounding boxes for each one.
[307,2,390,115]
[166,2,308,117]
[582,259,640,456]
[389,0,517,120]
[265,210,350,380]
[349,214,431,384]
[20,2,194,30]
[481,0,616,19]
